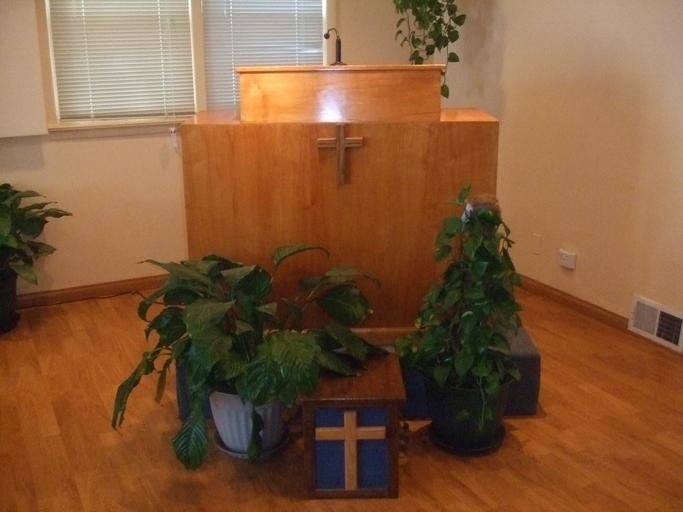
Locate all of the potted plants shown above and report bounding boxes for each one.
[391,175,528,455]
[111,241,389,471]
[0,183,73,334]
